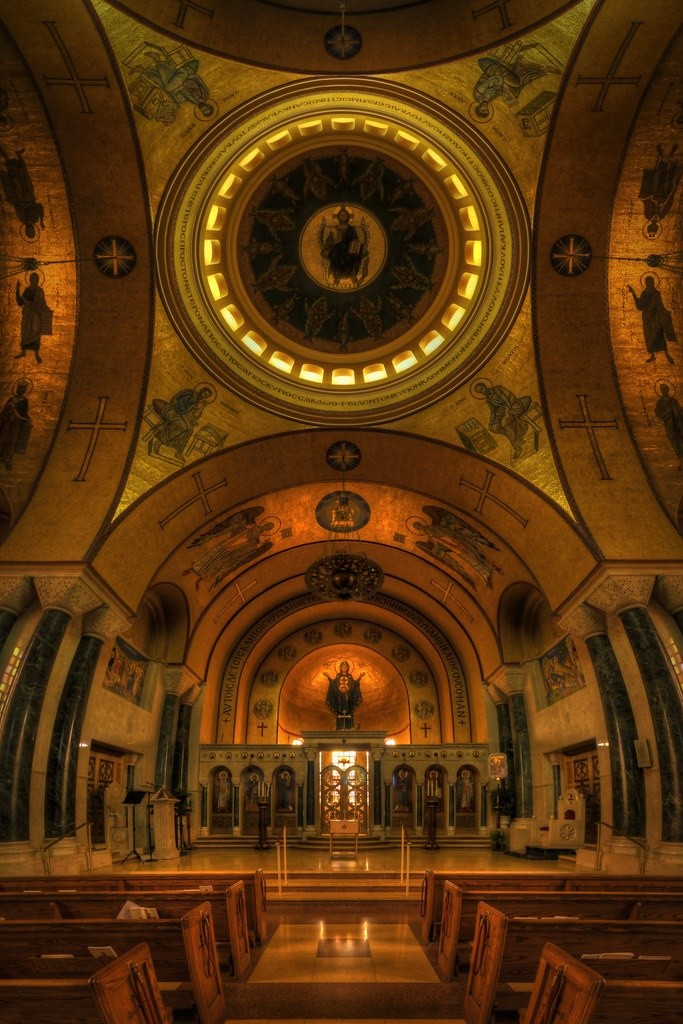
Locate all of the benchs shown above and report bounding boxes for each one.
[419,868,683,1024]
[0,867,265,1024]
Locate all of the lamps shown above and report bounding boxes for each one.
[487,752,509,852]
[303,441,385,603]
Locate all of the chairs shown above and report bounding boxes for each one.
[530,787,586,849]
